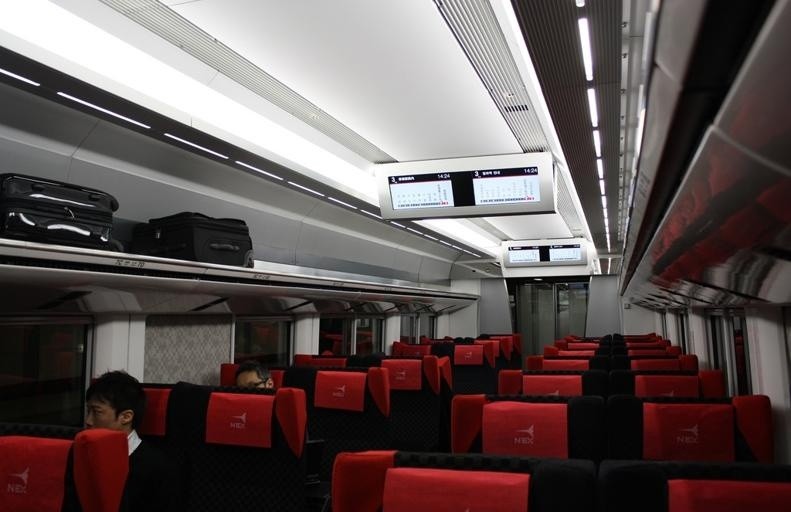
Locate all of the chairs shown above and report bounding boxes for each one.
[307,368,389,506]
[220,363,306,389]
[608,370,726,398]
[90,379,182,465]
[294,333,698,395]
[597,460,791,512]
[606,395,774,464]
[451,395,606,467]
[331,452,597,511]
[1,422,130,512]
[182,382,331,512]
[380,355,443,452]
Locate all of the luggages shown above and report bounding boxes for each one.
[133,211,254,269]
[0,173,124,251]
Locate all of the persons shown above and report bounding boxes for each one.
[234,360,274,390]
[83,372,171,512]
